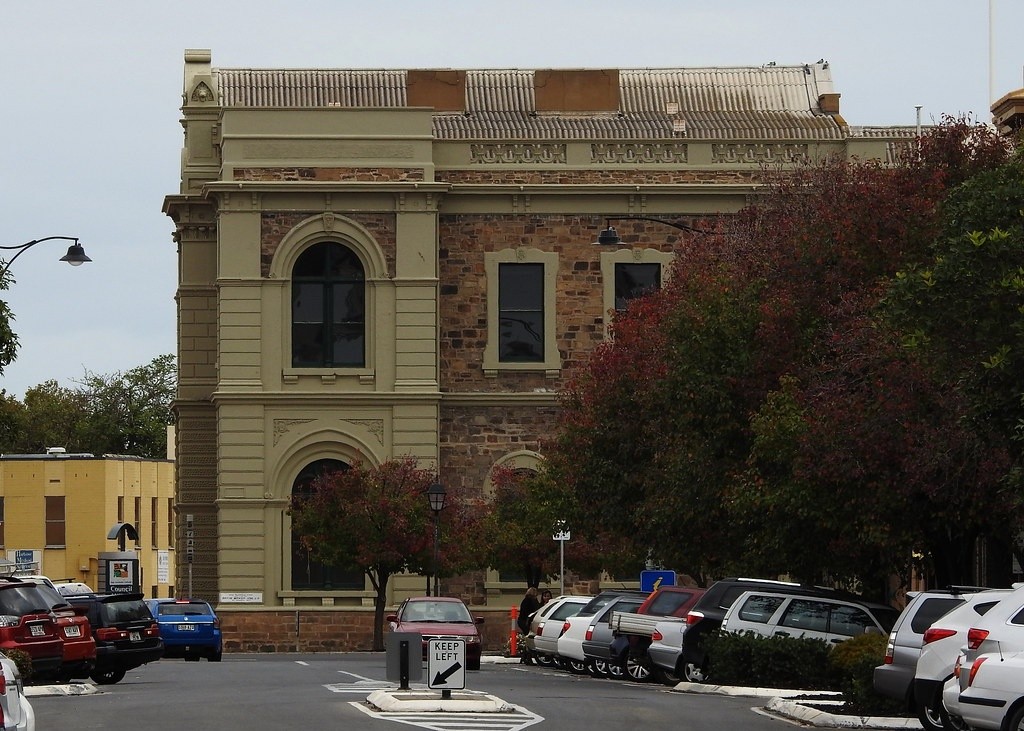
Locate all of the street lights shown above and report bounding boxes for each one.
[425,474,448,597]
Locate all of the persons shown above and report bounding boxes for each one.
[516,588,553,665]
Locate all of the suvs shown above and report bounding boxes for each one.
[683,577,870,687]
[718,590,899,649]
[914,588,1014,730]
[873,585,992,731]
[0,568,161,731]
[522,577,704,686]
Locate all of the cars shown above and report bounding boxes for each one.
[957,582,1024,731]
[386,595,486,670]
[144,597,223,661]
